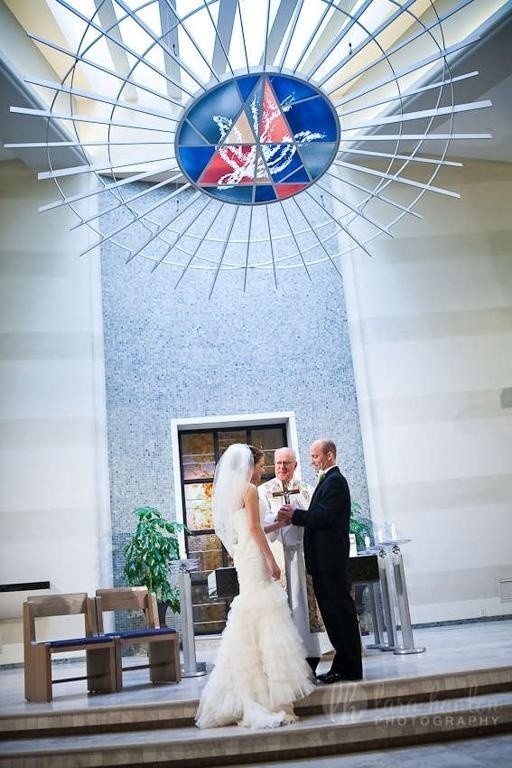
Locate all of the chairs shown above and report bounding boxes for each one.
[22,584,182,704]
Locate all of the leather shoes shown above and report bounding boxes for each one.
[315,668,346,684]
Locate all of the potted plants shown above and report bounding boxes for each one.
[345,497,372,557]
[117,507,195,629]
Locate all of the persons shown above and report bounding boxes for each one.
[257,444,317,678]
[274,440,364,685]
[194,442,318,730]
